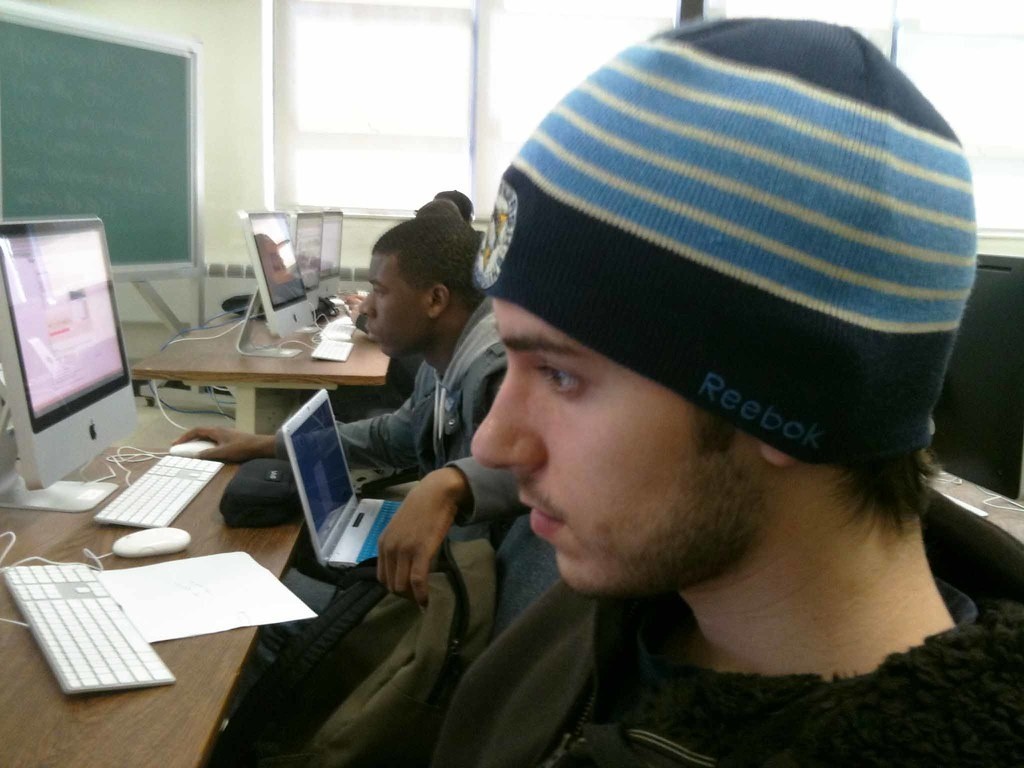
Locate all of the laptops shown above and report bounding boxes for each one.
[281,388,405,570]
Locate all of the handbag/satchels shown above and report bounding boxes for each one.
[219,459,303,528]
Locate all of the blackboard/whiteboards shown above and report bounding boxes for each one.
[0,4,209,285]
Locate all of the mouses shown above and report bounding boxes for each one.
[114,527,191,559]
[169,439,216,459]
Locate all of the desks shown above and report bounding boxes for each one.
[130,293,390,434]
[0,446,355,768]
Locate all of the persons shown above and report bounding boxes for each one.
[254,234,294,289]
[171,193,533,676]
[433,19,1024,768]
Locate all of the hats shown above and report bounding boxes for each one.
[471,17,975,463]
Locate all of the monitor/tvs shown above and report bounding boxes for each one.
[926,255,1024,500]
[0,216,137,512]
[236,210,344,359]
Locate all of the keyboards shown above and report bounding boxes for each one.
[312,316,357,362]
[93,456,224,529]
[1,564,176,695]
